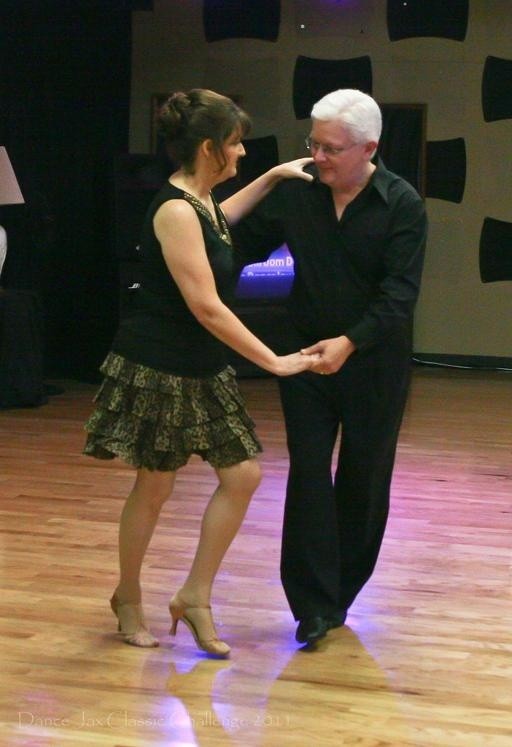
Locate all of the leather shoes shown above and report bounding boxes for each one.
[296,615,327,643]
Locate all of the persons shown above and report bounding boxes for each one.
[231,86,430,643]
[84,89,321,656]
[104,638,240,745]
[0,131,47,406]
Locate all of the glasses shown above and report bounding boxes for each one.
[304,136,353,155]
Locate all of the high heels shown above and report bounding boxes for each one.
[110,592,162,646]
[168,593,230,655]
